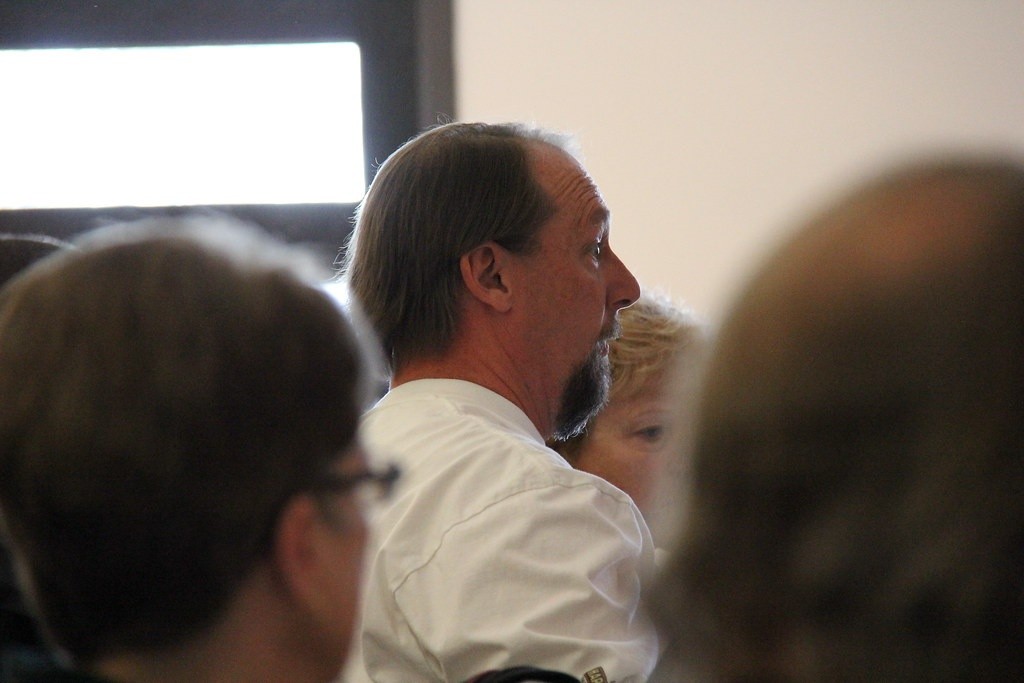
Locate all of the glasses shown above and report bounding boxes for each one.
[286,460,405,527]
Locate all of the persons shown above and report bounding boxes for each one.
[544,302,705,552]
[0,234,375,683]
[649,162,1023,683]
[336,122,680,683]
